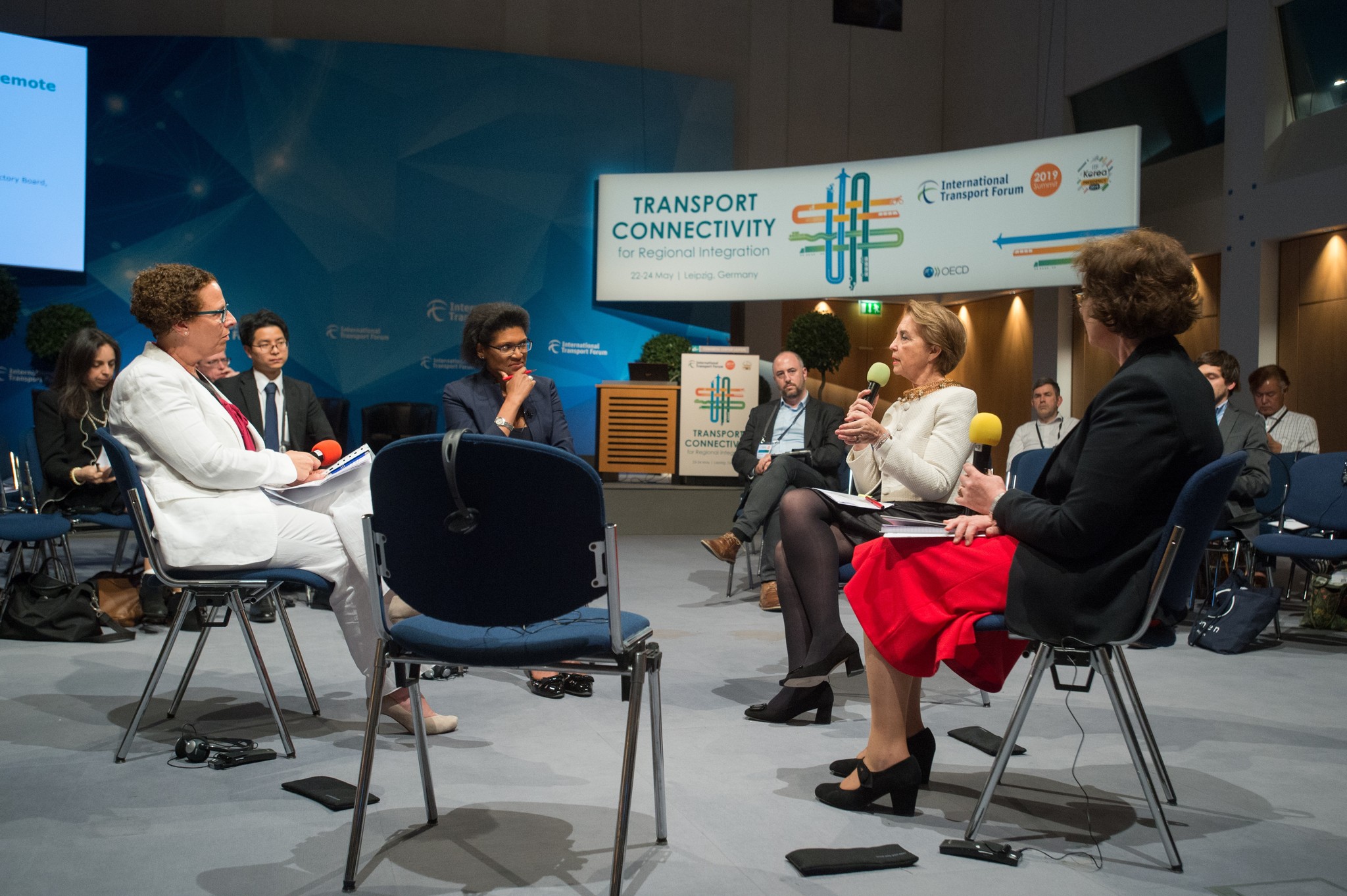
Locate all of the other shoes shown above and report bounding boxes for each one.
[170,593,203,631]
[139,574,169,625]
[1128,622,1177,649]
[1245,561,1267,585]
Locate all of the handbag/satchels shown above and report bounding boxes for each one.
[1298,564,1347,633]
[0,556,136,644]
[86,564,145,627]
[1188,568,1282,654]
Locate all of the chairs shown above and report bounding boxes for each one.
[362,401,440,456]
[31,388,136,572]
[342,434,669,896]
[0,451,78,593]
[91,427,333,764]
[314,397,350,458]
[726,449,1347,873]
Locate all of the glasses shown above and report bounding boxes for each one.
[250,340,290,353]
[484,339,533,357]
[1075,292,1092,307]
[182,303,230,323]
[204,357,231,368]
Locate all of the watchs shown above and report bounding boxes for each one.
[494,417,514,431]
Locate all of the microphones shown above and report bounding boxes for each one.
[525,411,533,418]
[969,412,1003,515]
[308,439,342,466]
[853,362,891,422]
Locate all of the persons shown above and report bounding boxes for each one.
[1248,364,1320,584]
[213,309,336,622]
[1127,349,1272,648]
[195,350,240,383]
[443,301,595,698]
[816,227,1223,815]
[1007,377,1080,472]
[700,350,846,610]
[107,264,459,735]
[745,299,978,724]
[34,328,201,632]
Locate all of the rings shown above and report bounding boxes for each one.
[854,435,857,442]
[528,375,533,381]
[957,487,962,496]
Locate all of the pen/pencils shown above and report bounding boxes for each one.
[503,368,538,381]
[866,496,884,509]
[326,450,369,478]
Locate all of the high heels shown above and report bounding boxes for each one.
[388,592,422,625]
[779,633,864,687]
[744,680,834,725]
[829,727,937,784]
[815,758,921,816]
[367,696,457,736]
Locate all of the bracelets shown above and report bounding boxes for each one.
[872,429,890,449]
[70,467,86,485]
[989,493,1005,520]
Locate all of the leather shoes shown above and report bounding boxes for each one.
[529,670,564,698]
[247,595,276,622]
[563,673,595,696]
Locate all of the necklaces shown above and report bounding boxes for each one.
[900,379,961,404]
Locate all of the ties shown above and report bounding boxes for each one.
[264,382,280,452]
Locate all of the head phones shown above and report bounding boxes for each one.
[442,427,482,535]
[420,664,468,680]
[174,733,254,764]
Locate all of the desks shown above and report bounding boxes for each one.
[595,385,681,484]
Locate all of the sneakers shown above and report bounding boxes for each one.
[700,531,745,565]
[759,580,781,610]
